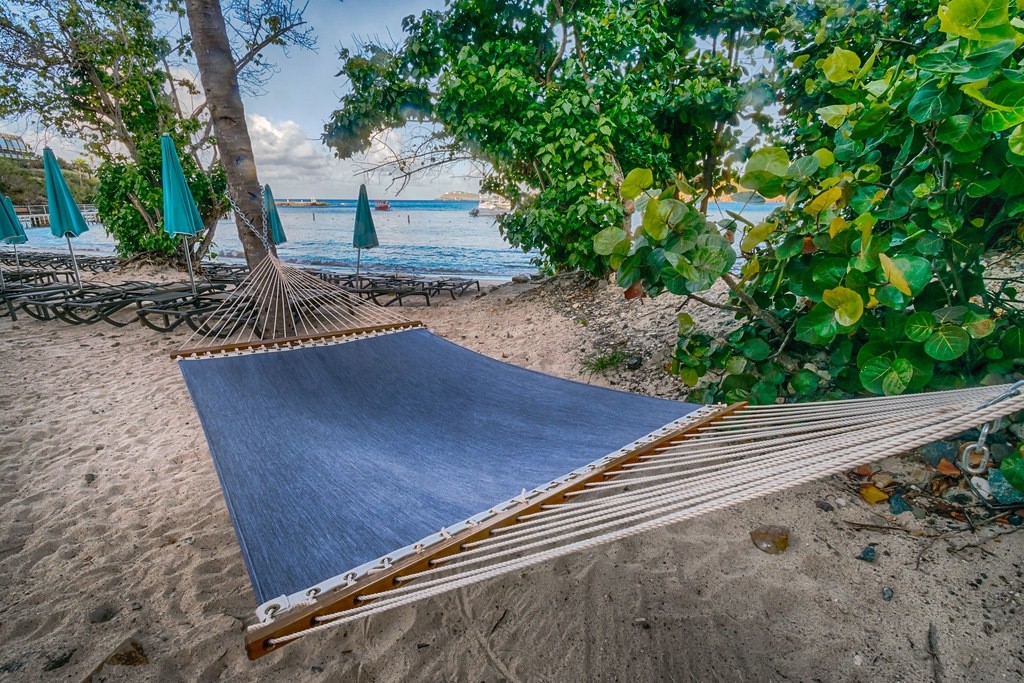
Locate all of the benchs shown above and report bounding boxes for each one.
[0,250,480,340]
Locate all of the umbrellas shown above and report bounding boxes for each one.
[0,192,24,287]
[264,184,287,245]
[4,196,29,290]
[352,183,379,287]
[43,145,89,296]
[161,132,206,296]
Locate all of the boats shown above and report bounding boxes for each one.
[374,202,392,211]
[478,202,496,209]
